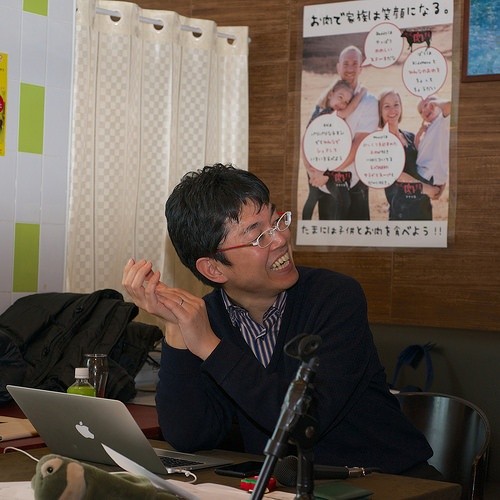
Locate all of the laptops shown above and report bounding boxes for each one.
[6,384,233,476]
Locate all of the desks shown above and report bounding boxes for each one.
[0,400,164,452]
[0,439,463,500]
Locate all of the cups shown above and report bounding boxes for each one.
[82,353,109,399]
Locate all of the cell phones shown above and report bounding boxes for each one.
[214,461,264,478]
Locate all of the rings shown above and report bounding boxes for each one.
[179,299,184,306]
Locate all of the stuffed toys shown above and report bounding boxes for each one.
[31,454,181,500]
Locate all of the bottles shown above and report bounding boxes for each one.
[66,368,96,397]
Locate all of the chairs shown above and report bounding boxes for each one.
[393,392,491,500]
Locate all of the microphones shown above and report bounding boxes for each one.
[274,455,372,487]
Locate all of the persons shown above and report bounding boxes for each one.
[302,45,450,221]
[121,163,444,480]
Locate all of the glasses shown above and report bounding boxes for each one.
[214,211,292,253]
